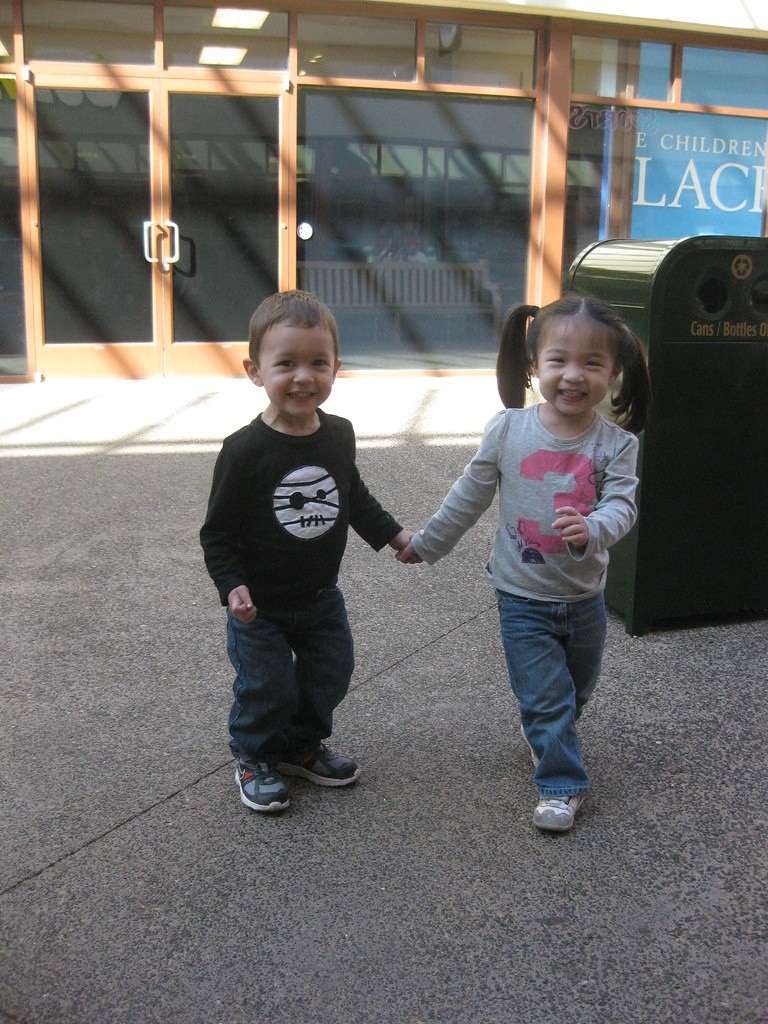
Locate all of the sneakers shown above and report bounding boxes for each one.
[235,763,291,812]
[520,723,539,768]
[532,793,583,831]
[279,742,359,786]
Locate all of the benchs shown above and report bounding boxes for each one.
[297,261,506,341]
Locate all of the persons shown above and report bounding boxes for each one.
[394,294,652,829]
[199,289,414,811]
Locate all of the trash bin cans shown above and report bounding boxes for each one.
[563,235,766,625]
